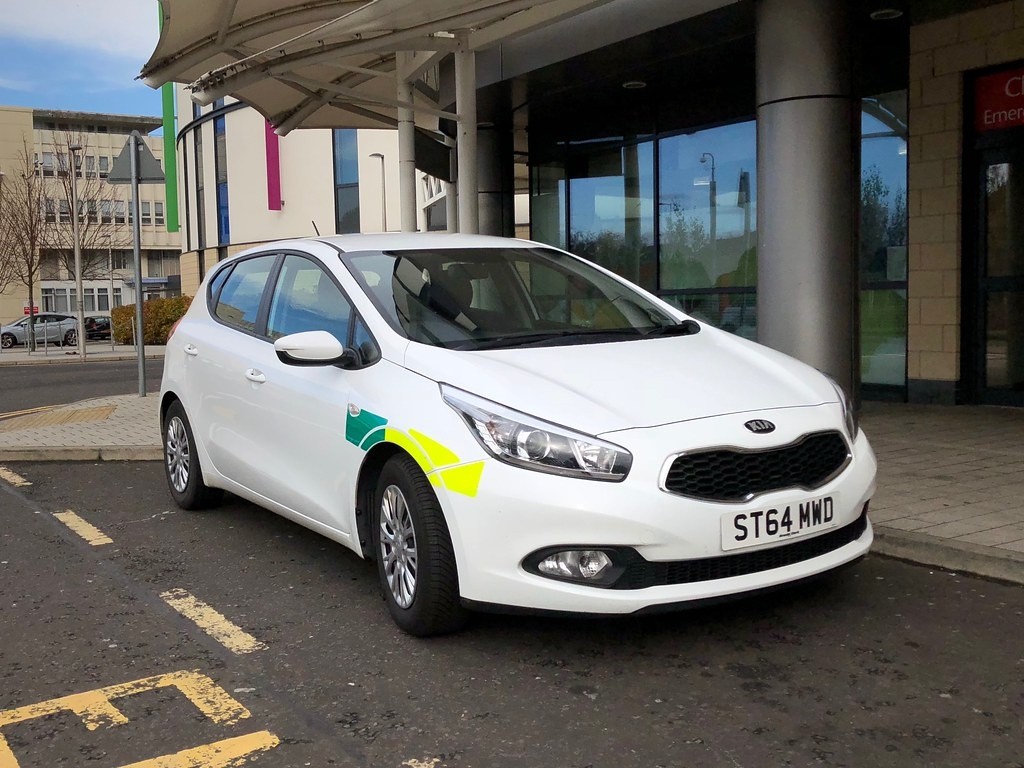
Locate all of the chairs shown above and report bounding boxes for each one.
[40,316,47,323]
[317,272,351,319]
[416,277,507,349]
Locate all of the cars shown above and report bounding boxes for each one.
[1,311,79,349]
[157,230,881,637]
[84,316,111,340]
